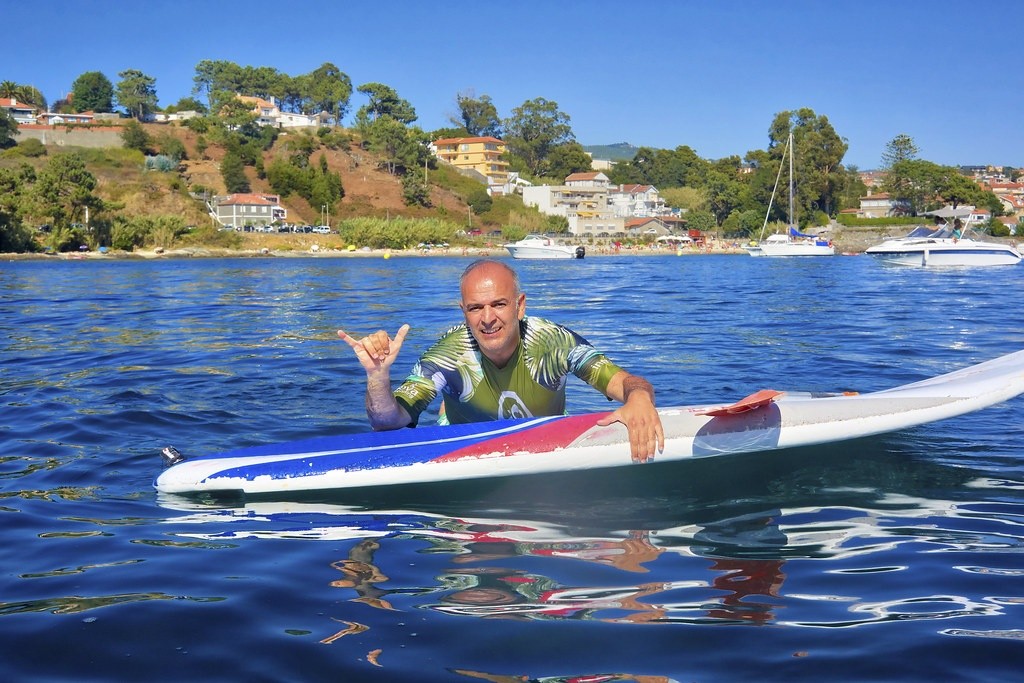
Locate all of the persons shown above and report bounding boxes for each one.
[334,256,666,464]
[925,215,946,231]
[948,218,964,238]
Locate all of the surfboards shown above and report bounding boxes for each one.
[153,350,1024,496]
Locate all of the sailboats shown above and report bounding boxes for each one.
[742,132,838,257]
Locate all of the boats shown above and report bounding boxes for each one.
[864,205,1023,268]
[504,234,587,260]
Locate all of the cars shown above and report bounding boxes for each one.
[217,222,331,234]
[39,223,50,233]
[489,230,503,237]
[468,227,484,237]
